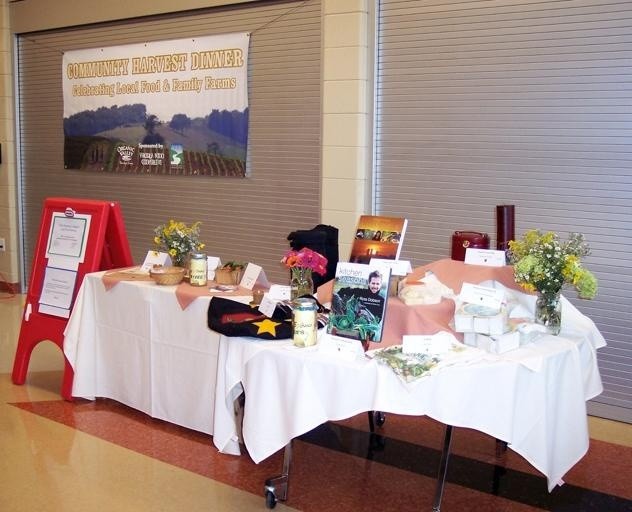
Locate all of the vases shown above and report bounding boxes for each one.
[170,254,189,273]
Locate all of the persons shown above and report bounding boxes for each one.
[372,231,381,241]
[338,270,384,324]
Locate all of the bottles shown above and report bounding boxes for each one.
[189,251,208,287]
[291,298,318,347]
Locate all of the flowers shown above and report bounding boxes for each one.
[281,247,328,294]
[151,219,206,265]
[504,227,598,325]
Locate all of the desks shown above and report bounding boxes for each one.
[65,265,304,451]
[220,281,608,509]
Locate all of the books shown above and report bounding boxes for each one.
[327,263,392,343]
[348,216,409,264]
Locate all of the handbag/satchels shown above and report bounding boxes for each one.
[288,224,338,295]
